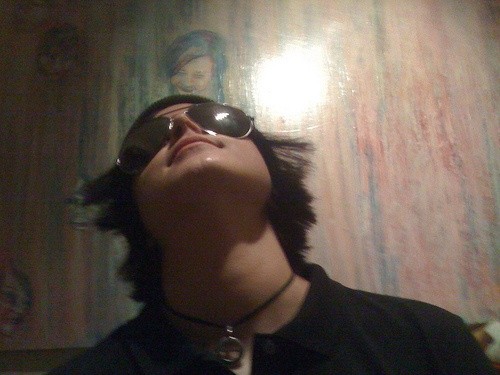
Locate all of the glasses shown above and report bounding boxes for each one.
[117,102,257,174]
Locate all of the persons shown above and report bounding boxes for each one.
[54,94,500,375]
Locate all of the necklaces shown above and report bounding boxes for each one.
[165,267,298,363]
